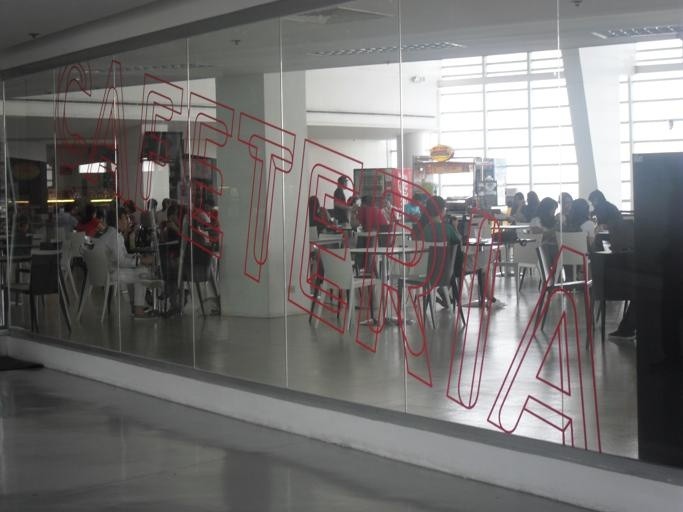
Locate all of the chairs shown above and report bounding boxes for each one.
[307,217,634,348]
[0,230,222,338]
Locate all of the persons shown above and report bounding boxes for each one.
[48,197,223,314]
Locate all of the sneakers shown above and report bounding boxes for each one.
[607,330,636,340]
[130,275,190,321]
